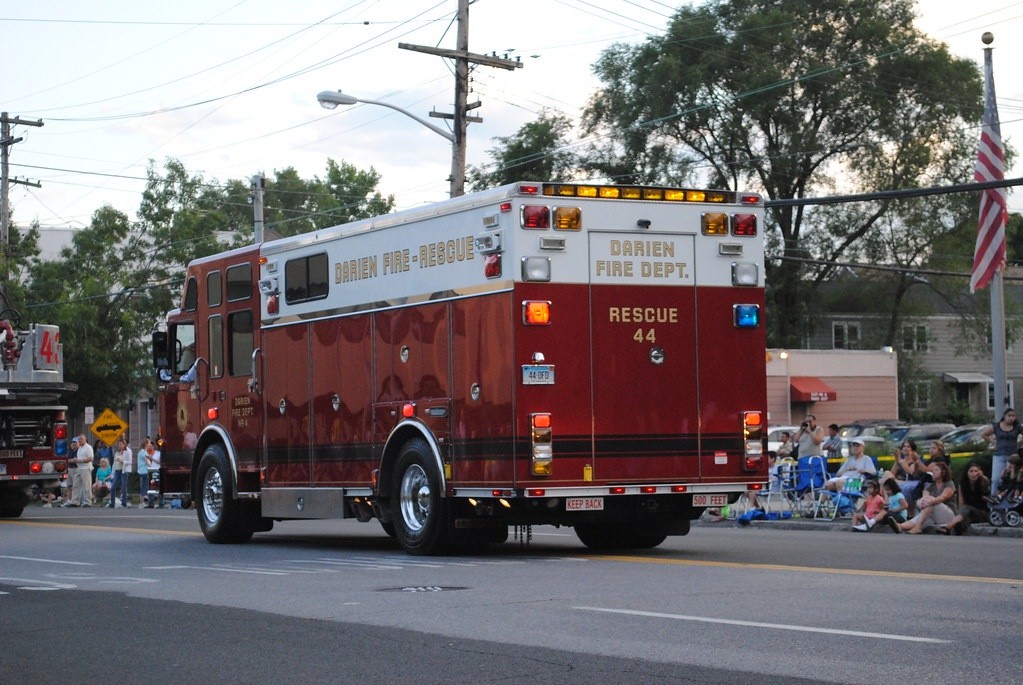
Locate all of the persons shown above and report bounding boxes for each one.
[42,434,195,511]
[697,407,1023,534]
[171,361,197,383]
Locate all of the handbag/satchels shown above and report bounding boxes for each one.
[100,481,109,498]
[789,445,800,460]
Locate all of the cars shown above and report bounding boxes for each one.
[747,426,801,457]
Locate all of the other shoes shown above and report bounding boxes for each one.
[33,493,196,510]
[861,513,874,530]
[806,511,822,519]
[906,528,922,534]
[1011,497,1021,503]
[826,509,841,518]
[888,517,901,534]
[851,525,868,532]
[988,495,1001,504]
[932,523,952,535]
[1006,497,1016,505]
[983,495,998,506]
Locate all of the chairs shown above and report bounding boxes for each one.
[814,455,881,522]
[737,457,833,520]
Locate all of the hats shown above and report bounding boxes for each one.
[71,437,78,443]
[850,439,866,447]
[1007,455,1021,467]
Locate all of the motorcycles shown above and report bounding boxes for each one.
[821,420,992,477]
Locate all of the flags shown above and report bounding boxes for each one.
[967,50,1007,293]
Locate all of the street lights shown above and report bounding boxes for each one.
[317,91,470,199]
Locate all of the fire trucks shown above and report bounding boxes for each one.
[154,181,770,554]
[0,318,68,517]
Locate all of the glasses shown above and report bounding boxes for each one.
[851,442,862,447]
